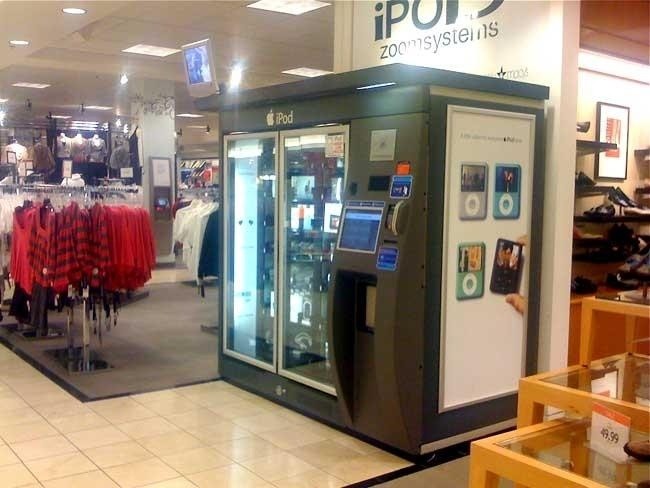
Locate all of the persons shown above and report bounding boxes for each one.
[498,243,518,269]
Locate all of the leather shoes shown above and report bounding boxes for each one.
[583,233,603,239]
[593,205,615,218]
[606,273,638,290]
[636,186,650,194]
[574,276,597,295]
[617,243,650,276]
[577,126,581,130]
[624,206,650,216]
[584,207,597,216]
[578,121,590,132]
[637,259,650,279]
[624,439,650,462]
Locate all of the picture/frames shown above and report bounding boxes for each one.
[594,101,629,179]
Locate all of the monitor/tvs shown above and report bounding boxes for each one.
[321,200,342,235]
[288,200,317,234]
[336,200,384,255]
[181,38,219,99]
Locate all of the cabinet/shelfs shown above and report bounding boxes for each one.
[469,415,650,488]
[518,351,649,427]
[579,294,650,366]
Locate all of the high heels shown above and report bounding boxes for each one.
[608,186,628,207]
[578,172,596,186]
[616,187,638,207]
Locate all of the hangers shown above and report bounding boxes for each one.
[1,183,143,242]
[178,189,222,226]
